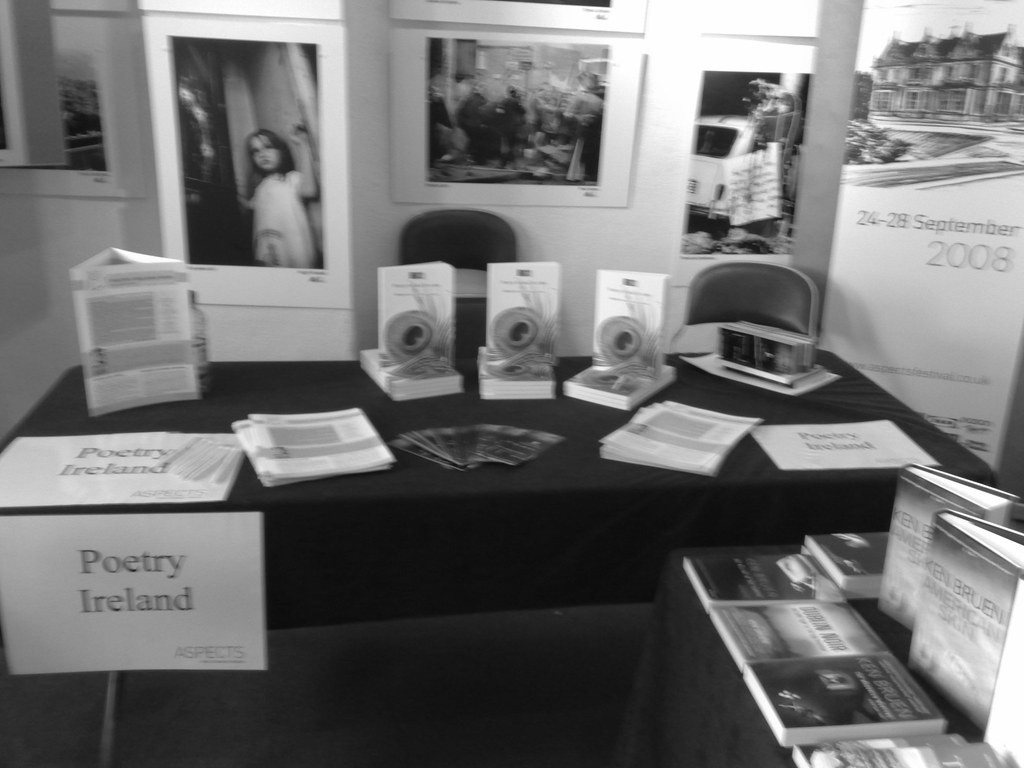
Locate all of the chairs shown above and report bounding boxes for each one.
[671,264,819,350]
[401,211,517,357]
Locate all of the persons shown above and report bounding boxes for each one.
[233,124,320,269]
[428,67,602,185]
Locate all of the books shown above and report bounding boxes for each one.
[682,462,1024,768]
[357,260,676,412]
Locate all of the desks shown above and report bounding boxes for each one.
[623,543,984,768]
[0,350,995,768]
